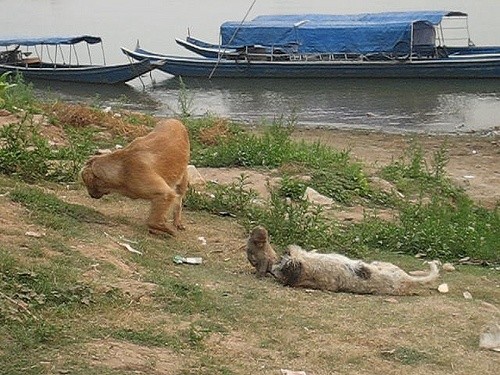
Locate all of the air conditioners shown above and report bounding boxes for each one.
[272,243,439,296]
[79,118,191,239]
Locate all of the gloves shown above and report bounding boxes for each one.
[121,39,500,79]
[0,35,167,86]
[174,10,500,61]
